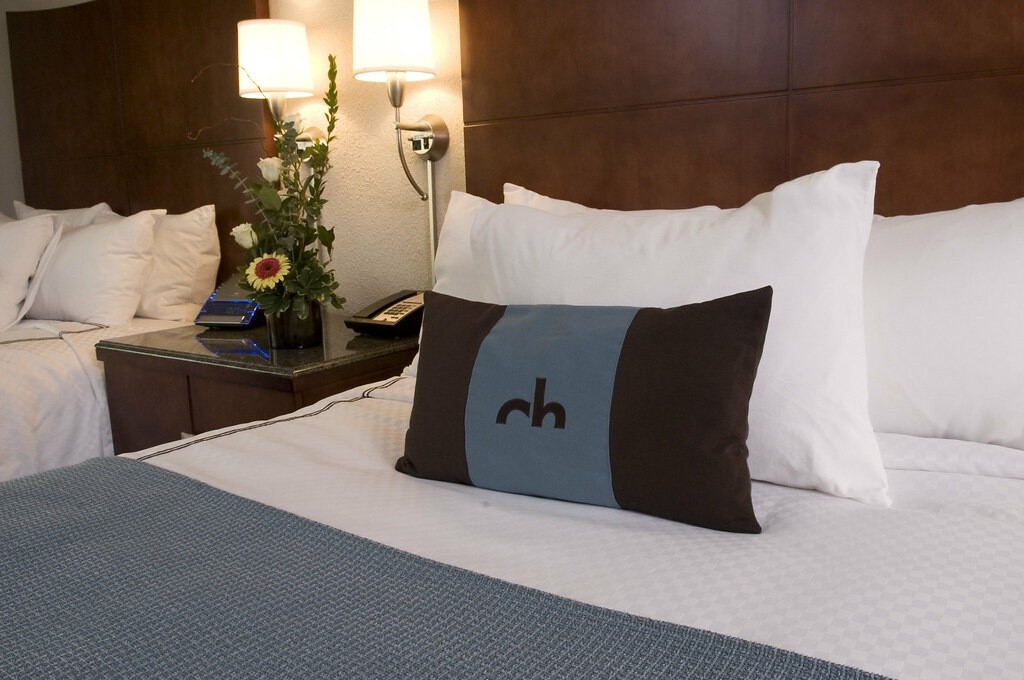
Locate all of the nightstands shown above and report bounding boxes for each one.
[95,321,420,456]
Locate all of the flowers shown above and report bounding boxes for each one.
[187,54,346,318]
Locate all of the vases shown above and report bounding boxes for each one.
[262,298,323,348]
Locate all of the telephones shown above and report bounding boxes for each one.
[343,288,424,336]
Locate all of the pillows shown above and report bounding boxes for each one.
[0,213,66,329]
[400,160,892,507]
[131,204,221,323]
[14,201,109,231]
[396,286,772,534]
[865,198,1024,453]
[23,208,167,329]
[502,182,619,215]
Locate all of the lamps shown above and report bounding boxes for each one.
[353,0,449,288]
[237,19,330,264]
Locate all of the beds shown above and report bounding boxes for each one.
[0,0,283,481]
[0,0,1024,680]
